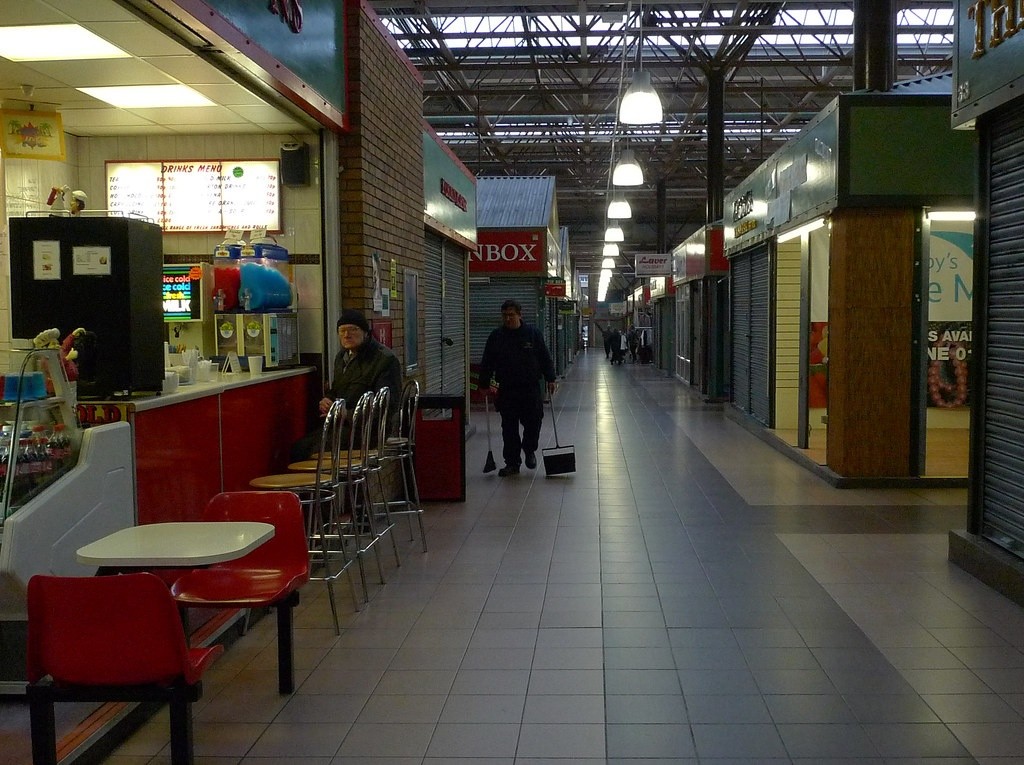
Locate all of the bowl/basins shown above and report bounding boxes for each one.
[167,368,191,381]
[158,374,179,393]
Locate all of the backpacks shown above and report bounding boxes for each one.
[631,330,639,345]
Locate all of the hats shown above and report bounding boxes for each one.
[337,309,369,334]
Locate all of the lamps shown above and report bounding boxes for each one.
[619,0,662,124]
[613,124,644,186]
[597,191,632,302]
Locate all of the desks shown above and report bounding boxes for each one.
[76,522,272,641]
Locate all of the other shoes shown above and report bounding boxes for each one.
[618,361,622,365]
[355,518,371,534]
[308,529,334,548]
[521,444,536,469]
[610,360,613,365]
[498,461,521,476]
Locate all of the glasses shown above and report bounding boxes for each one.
[503,312,519,317]
[338,326,361,336]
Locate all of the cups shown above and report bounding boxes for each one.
[0,372,47,401]
[247,356,263,374]
[210,364,219,381]
[183,360,198,379]
[199,367,210,381]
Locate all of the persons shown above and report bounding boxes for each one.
[70,190,88,217]
[478,300,556,476]
[33,328,87,394]
[50,185,70,216]
[293,313,401,558]
[602,326,638,365]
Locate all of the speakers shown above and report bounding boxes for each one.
[280,141,311,188]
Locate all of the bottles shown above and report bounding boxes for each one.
[9,338,37,371]
[0,420,69,506]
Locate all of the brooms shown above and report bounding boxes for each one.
[483,393,497,474]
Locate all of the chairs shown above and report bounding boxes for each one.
[26,379,430,765]
